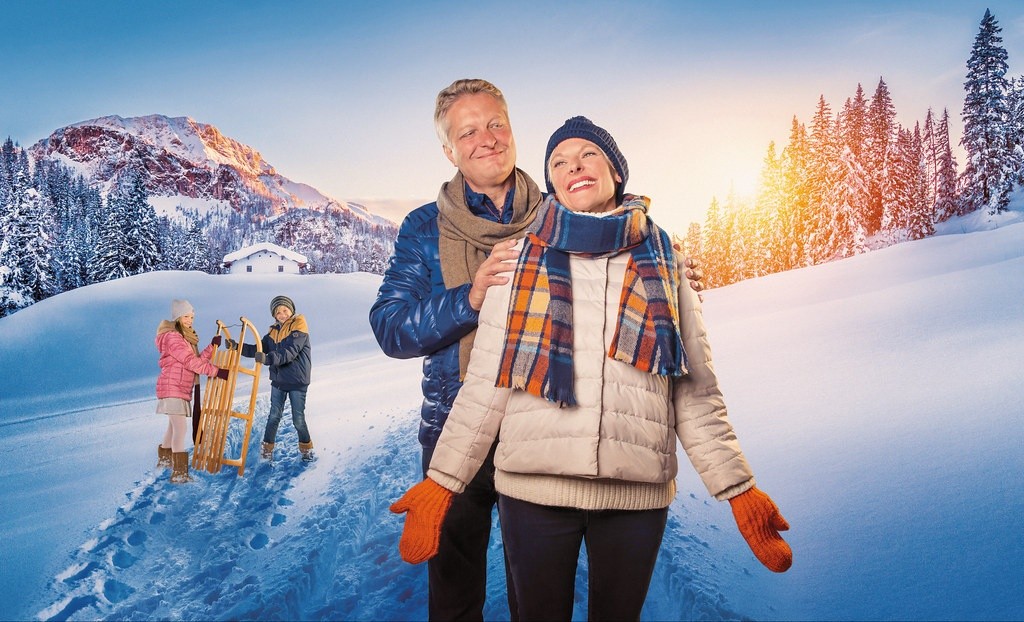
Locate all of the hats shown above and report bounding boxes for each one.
[270,296,295,317]
[544,115,629,208]
[171,300,194,322]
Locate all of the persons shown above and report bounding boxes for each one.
[368,79,548,622]
[154,299,229,483]
[224,296,316,461]
[389,116,793,622]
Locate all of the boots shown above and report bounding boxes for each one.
[156,444,172,468]
[261,440,275,464]
[170,452,194,483]
[299,440,314,464]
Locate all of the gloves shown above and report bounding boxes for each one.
[211,335,222,347]
[217,368,229,381]
[390,475,455,565]
[255,352,270,365]
[728,485,793,573]
[225,339,237,350]
